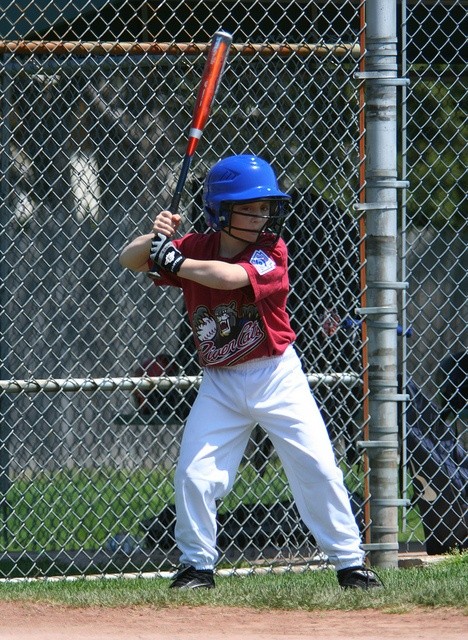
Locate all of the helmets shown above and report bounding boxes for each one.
[135,356,181,414]
[201,154,292,249]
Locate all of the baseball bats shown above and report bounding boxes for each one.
[146,31,234,281]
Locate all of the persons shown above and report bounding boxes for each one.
[120,153,385,594]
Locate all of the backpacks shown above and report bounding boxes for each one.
[397,371,468,553]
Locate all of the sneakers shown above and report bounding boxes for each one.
[337,562,383,590]
[168,565,214,590]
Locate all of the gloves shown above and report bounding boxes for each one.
[144,234,184,280]
